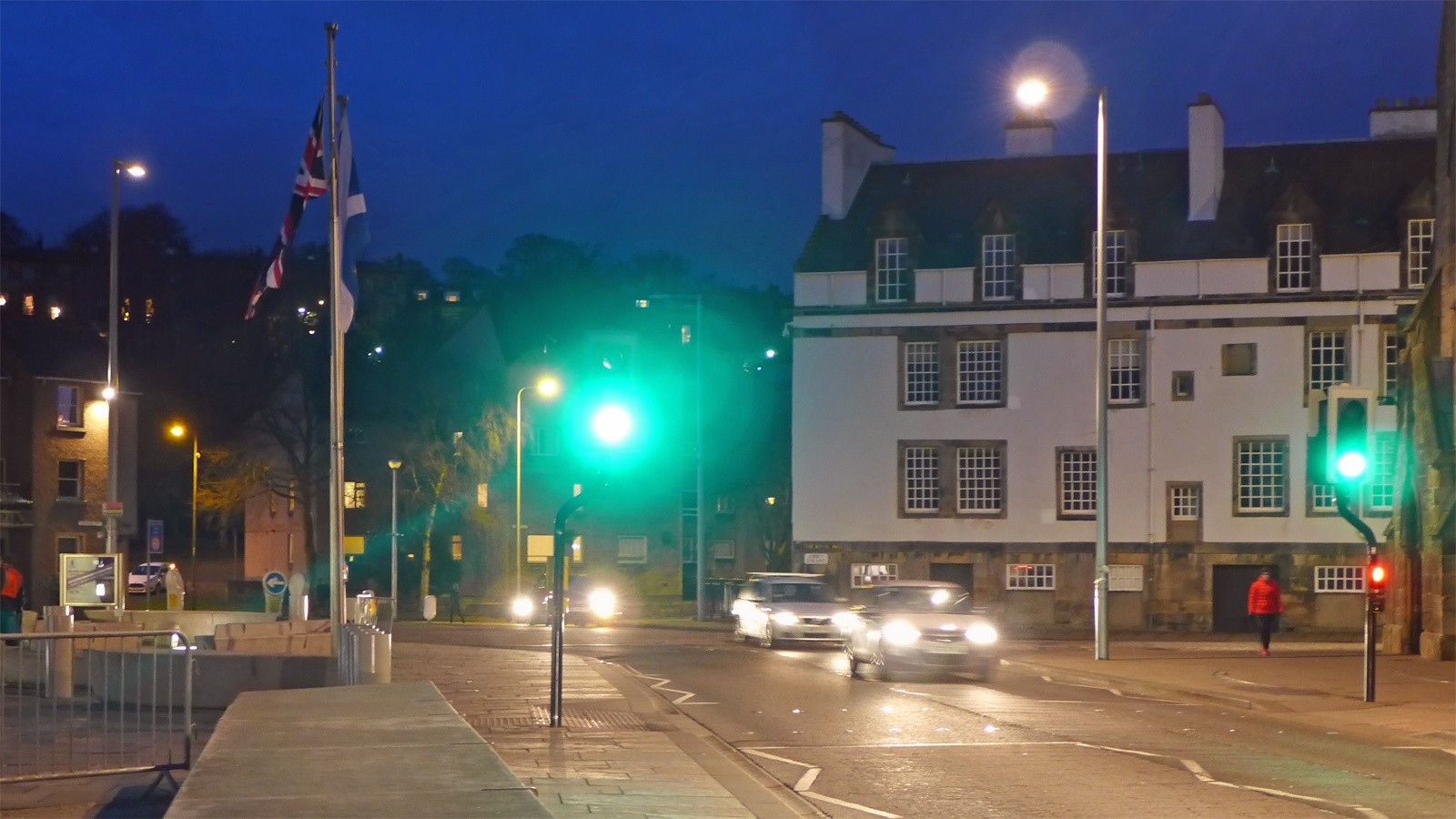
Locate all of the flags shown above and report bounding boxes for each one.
[241,86,329,328]
[332,115,373,334]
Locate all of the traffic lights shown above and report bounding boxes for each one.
[1327,387,1377,483]
[1364,565,1385,614]
[571,379,658,468]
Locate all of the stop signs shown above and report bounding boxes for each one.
[165,570,185,611]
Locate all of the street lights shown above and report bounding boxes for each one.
[1015,76,1108,659]
[171,426,201,609]
[387,459,402,619]
[517,376,562,598]
[105,157,147,554]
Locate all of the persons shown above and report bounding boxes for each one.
[1248,568,1284,659]
[449,583,466,624]
[0,558,24,648]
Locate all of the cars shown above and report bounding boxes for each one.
[732,572,862,649]
[128,561,171,596]
[844,581,1002,683]
[509,570,622,628]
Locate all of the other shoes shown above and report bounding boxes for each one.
[1262,647,1270,657]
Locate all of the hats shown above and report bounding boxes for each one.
[1260,567,1271,575]
